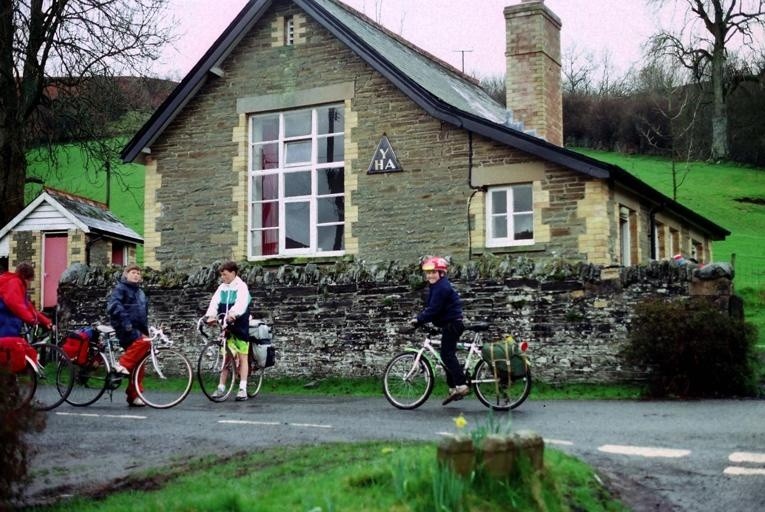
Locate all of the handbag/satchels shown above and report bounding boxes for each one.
[482,335,525,385]
[63,326,103,372]
[248,319,275,368]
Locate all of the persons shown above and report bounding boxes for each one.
[407,257,470,405]
[107,264,152,406]
[205,260,252,400]
[0,263,52,373]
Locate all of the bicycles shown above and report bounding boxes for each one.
[14,321,75,412]
[0,346,45,416]
[196,314,266,402]
[380,321,531,412]
[56,324,192,410]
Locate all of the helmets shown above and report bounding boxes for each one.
[422,257,448,275]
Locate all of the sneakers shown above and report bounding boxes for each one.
[113,361,130,374]
[442,385,469,405]
[129,397,145,407]
[210,390,224,398]
[235,389,248,401]
